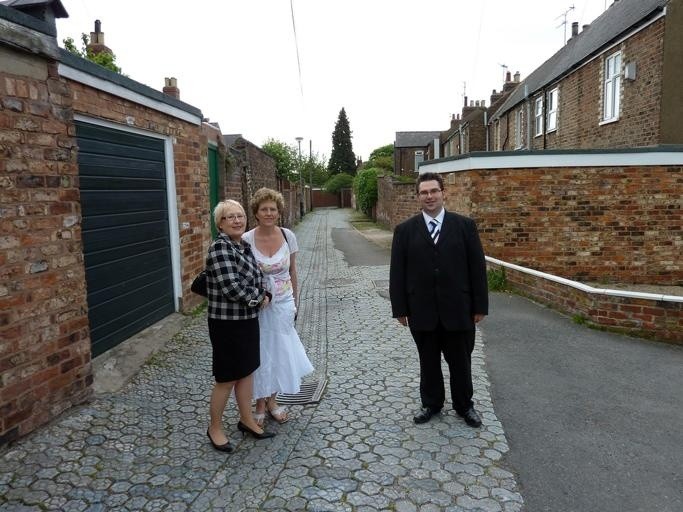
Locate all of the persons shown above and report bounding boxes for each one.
[242,188,315,428]
[204,200,276,452]
[387,172,488,428]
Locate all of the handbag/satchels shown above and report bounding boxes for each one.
[190,269,207,297]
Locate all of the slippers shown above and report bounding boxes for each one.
[266,406,290,425]
[254,412,265,429]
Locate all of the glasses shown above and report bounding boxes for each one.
[418,188,442,196]
[221,213,245,221]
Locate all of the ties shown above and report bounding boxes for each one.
[428,218,440,247]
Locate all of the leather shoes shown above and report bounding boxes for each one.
[456,406,481,428]
[413,403,443,424]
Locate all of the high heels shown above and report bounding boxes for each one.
[207,427,232,452]
[237,421,276,439]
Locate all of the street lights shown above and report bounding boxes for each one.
[295,137,305,223]
